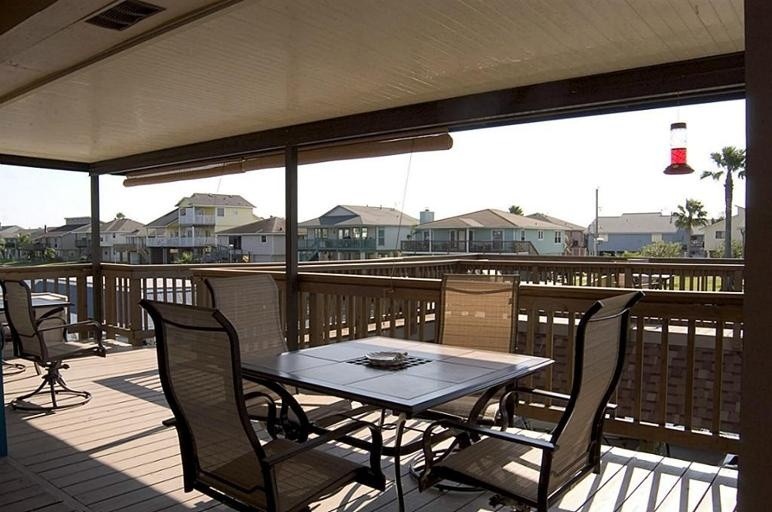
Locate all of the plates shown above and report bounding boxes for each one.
[366,350,410,367]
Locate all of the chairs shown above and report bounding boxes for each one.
[138,272,643,511]
[0,278,108,416]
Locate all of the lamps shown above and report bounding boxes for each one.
[663,95,695,176]
